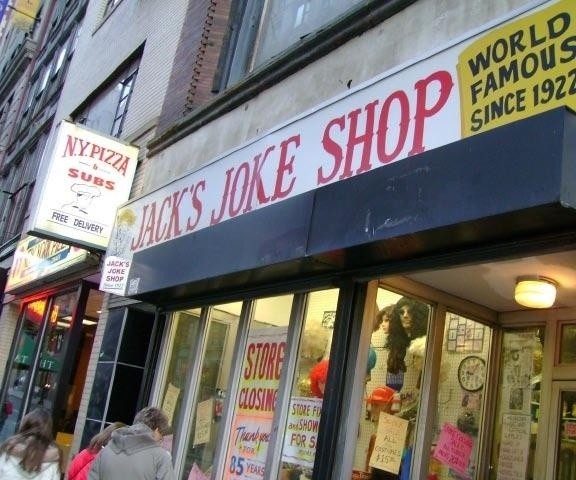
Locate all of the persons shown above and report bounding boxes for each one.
[67,422,127,480]
[0,409,61,480]
[296,327,329,399]
[85,406,179,480]
[367,295,430,422]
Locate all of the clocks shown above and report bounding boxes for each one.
[456,354,486,394]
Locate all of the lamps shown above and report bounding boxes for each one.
[513,272,562,309]
